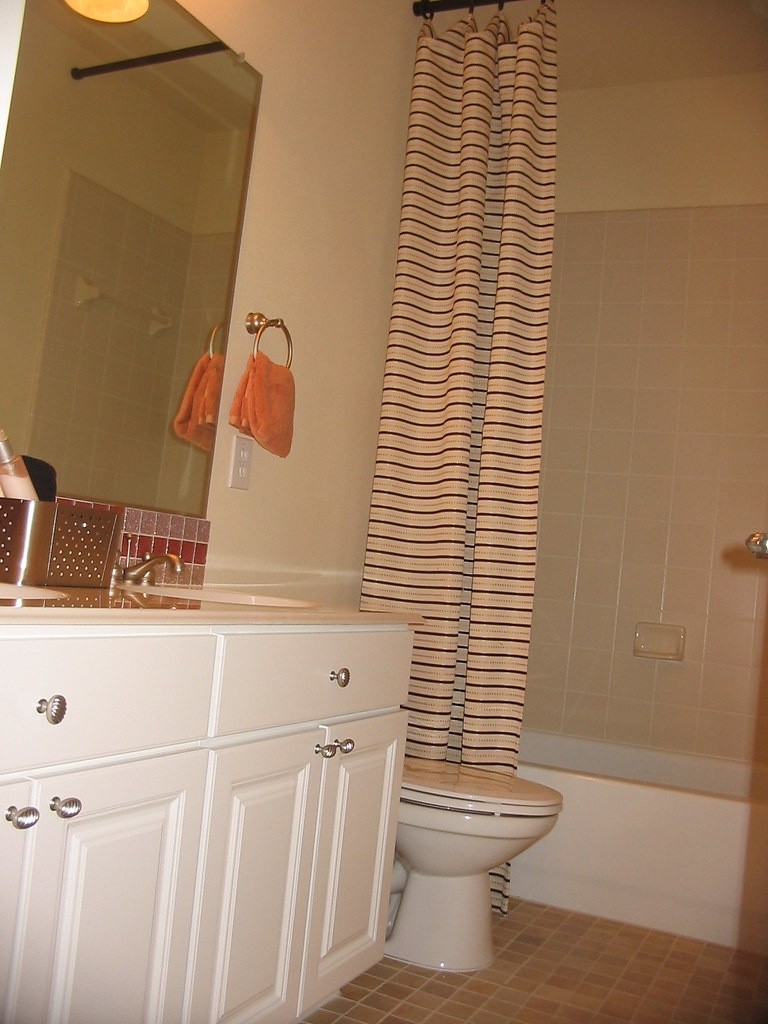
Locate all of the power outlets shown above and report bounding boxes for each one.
[228,436,256,492]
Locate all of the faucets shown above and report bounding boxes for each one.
[123,554,183,580]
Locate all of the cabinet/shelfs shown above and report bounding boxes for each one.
[0,745,209,1024]
[184,711,407,1024]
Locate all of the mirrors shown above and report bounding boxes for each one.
[0,0,264,515]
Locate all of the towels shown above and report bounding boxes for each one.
[173,352,226,454]
[227,350,295,459]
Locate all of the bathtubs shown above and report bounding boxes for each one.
[504,728,768,957]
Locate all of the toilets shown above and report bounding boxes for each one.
[383,757,565,973]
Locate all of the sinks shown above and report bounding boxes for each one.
[115,586,318,607]
[0,582,69,600]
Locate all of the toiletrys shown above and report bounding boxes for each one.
[0,427,39,501]
